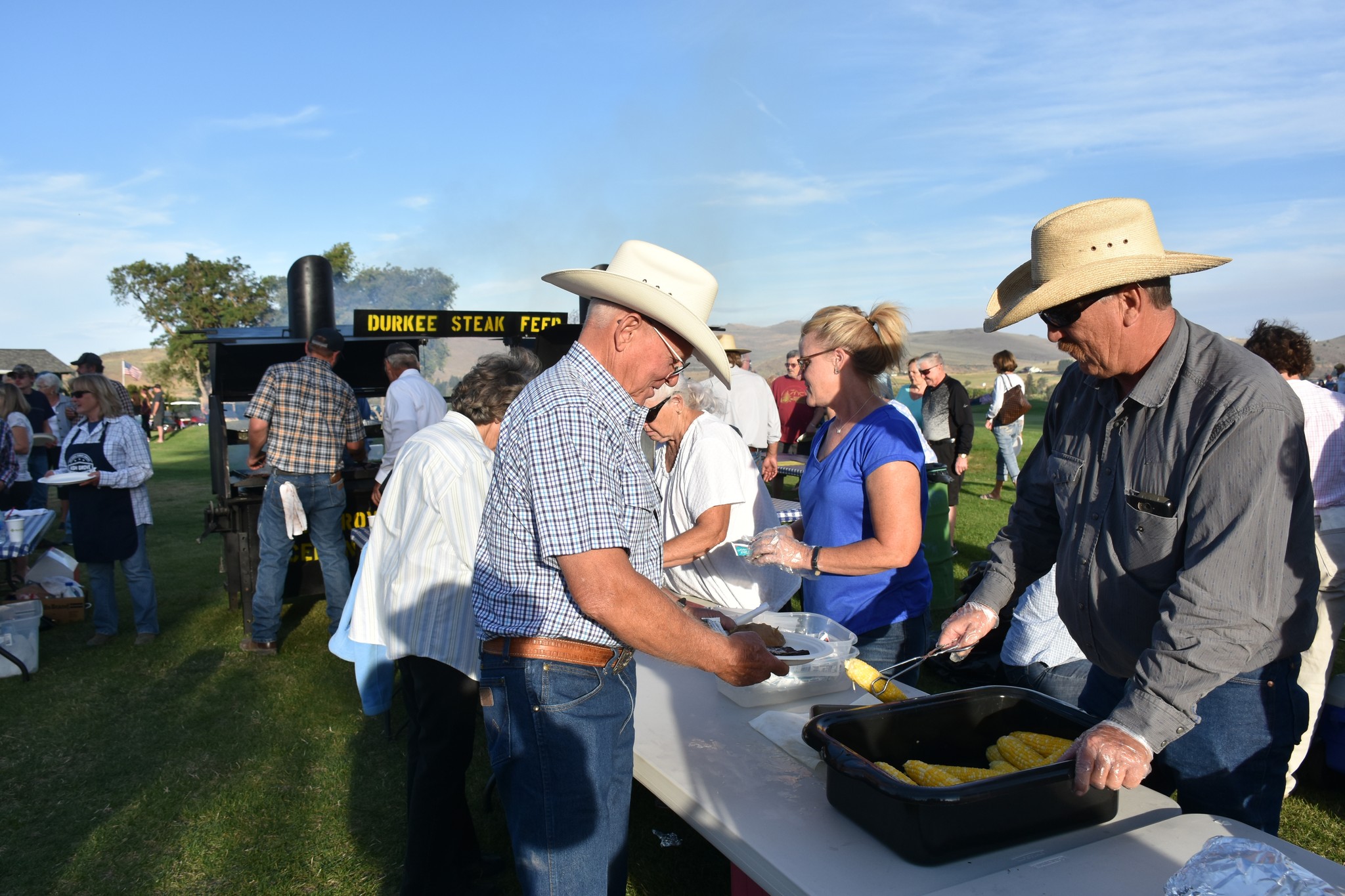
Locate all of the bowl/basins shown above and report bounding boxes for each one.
[709,606,860,708]
[802,684,1119,867]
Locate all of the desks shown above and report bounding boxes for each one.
[0,510,57,679]
[761,452,809,499]
[772,498,802,522]
[632,623,1183,896]
[917,813,1345,896]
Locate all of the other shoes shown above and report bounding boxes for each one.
[134,632,155,644]
[238,637,278,654]
[36,538,60,549]
[85,633,116,646]
[790,480,801,491]
[57,522,65,531]
[950,542,958,556]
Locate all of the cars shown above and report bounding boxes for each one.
[1017,367,1042,374]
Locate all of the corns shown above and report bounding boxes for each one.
[844,658,908,703]
[985,744,1007,761]
[989,760,1020,773]
[997,736,1045,770]
[873,762,918,785]
[929,764,1008,782]
[1008,732,1075,757]
[1030,745,1070,768]
[902,760,964,786]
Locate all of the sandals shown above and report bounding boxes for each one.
[980,493,1000,501]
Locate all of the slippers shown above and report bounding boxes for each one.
[1,574,25,588]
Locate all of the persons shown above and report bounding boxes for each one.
[893,357,928,436]
[916,351,975,555]
[372,342,449,508]
[688,334,835,482]
[372,346,546,895]
[470,262,786,896]
[1242,318,1345,799]
[640,368,797,615]
[0,352,166,599]
[44,374,159,650]
[748,297,932,689]
[1318,380,1325,388]
[959,217,1318,837]
[979,350,1025,502]
[239,326,364,652]
[1325,375,1334,384]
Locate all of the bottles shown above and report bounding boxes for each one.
[63,582,77,598]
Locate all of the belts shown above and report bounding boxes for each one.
[747,446,762,452]
[927,437,957,445]
[481,630,637,675]
[271,467,311,476]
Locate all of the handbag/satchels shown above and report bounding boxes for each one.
[996,373,1032,426]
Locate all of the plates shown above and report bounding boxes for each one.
[724,629,834,666]
[37,472,97,485]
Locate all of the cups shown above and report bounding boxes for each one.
[367,444,384,462]
[367,515,375,532]
[4,518,25,542]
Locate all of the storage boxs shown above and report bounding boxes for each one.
[716,613,1119,867]
[0,547,92,678]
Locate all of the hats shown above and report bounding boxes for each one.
[1325,375,1331,378]
[7,364,35,379]
[152,384,161,388]
[541,237,753,392]
[983,197,1234,334]
[70,353,104,368]
[311,327,418,357]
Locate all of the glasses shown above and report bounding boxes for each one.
[643,392,676,424]
[1034,290,1113,327]
[741,344,861,372]
[617,315,692,379]
[918,363,943,375]
[11,374,24,380]
[70,390,94,398]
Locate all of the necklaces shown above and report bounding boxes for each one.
[834,393,875,433]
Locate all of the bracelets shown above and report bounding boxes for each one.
[809,423,816,428]
[958,453,968,459]
[811,545,821,576]
[986,417,994,420]
